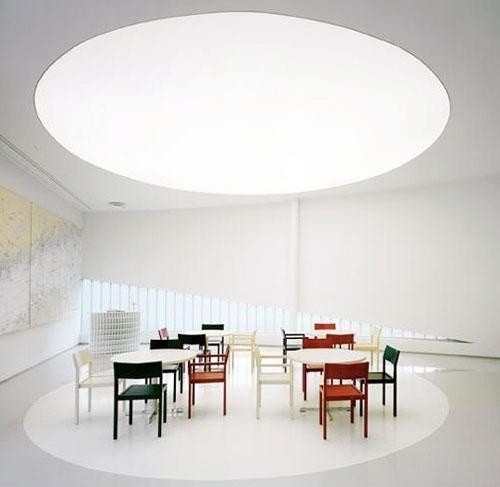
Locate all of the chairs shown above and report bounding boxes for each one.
[231,328,255,366]
[253,347,295,420]
[113,362,168,440]
[178,333,212,372]
[319,361,369,440]
[314,323,337,339]
[353,324,381,370]
[72,349,127,426]
[188,344,231,418]
[158,327,169,340]
[360,345,400,416]
[325,333,355,349]
[280,327,308,372]
[201,324,224,361]
[302,339,334,401]
[150,339,185,404]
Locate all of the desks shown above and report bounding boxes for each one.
[309,329,355,339]
[110,349,196,424]
[175,330,231,363]
[289,348,367,408]
